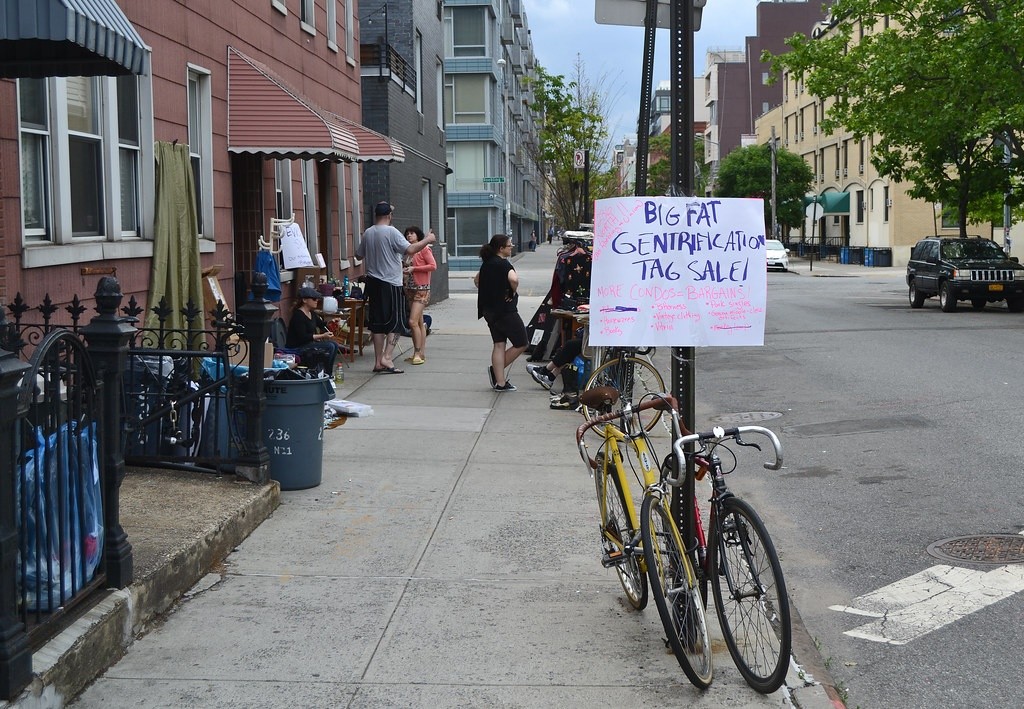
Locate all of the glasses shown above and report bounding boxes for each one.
[308,297,317,301]
[504,244,511,247]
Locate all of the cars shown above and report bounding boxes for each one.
[765,238,791,272]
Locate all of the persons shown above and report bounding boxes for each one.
[400,226,438,365]
[526,328,584,389]
[548,227,553,244]
[355,201,436,373]
[474,234,528,391]
[531,230,537,242]
[556,230,561,240]
[284,287,338,378]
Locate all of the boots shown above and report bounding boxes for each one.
[550,363,582,409]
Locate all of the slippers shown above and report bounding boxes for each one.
[373,367,388,372]
[404,355,427,361]
[412,358,425,364]
[379,365,404,373]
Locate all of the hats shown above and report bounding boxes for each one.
[297,287,322,299]
[376,201,395,216]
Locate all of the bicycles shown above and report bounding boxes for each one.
[559,303,793,694]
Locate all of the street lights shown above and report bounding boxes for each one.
[496,57,513,261]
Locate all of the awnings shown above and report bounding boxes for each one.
[801,192,850,219]
[227,46,405,163]
[0,0,147,79]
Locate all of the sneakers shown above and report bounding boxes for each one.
[526,363,542,375]
[488,365,497,388]
[494,379,517,391]
[531,370,553,390]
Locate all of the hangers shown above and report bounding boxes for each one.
[557,240,592,264]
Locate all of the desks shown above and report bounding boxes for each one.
[318,300,366,361]
[549,312,589,349]
[314,309,352,368]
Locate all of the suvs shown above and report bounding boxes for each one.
[905,235,1024,314]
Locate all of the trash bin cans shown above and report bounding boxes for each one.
[123,348,171,463]
[864,247,891,267]
[201,357,290,472]
[840,247,863,264]
[162,354,194,462]
[18,370,69,455]
[236,368,335,491]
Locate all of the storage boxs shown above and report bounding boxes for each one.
[230,337,274,368]
[201,264,232,320]
[293,267,320,298]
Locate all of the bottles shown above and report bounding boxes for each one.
[343,276,350,297]
[335,363,344,384]
